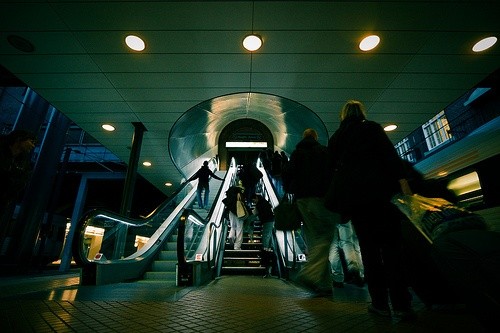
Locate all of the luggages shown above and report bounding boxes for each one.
[399,216,500,312]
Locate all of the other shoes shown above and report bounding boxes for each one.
[368,304,391,316]
[352,271,366,287]
[331,282,344,290]
[318,290,329,300]
[391,309,414,321]
[299,280,319,292]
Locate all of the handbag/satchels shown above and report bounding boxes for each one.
[403,193,487,241]
[324,175,357,217]
[237,193,249,219]
[274,192,301,232]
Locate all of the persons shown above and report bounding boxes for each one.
[0,131,37,229]
[188,162,225,209]
[222,100,417,321]
